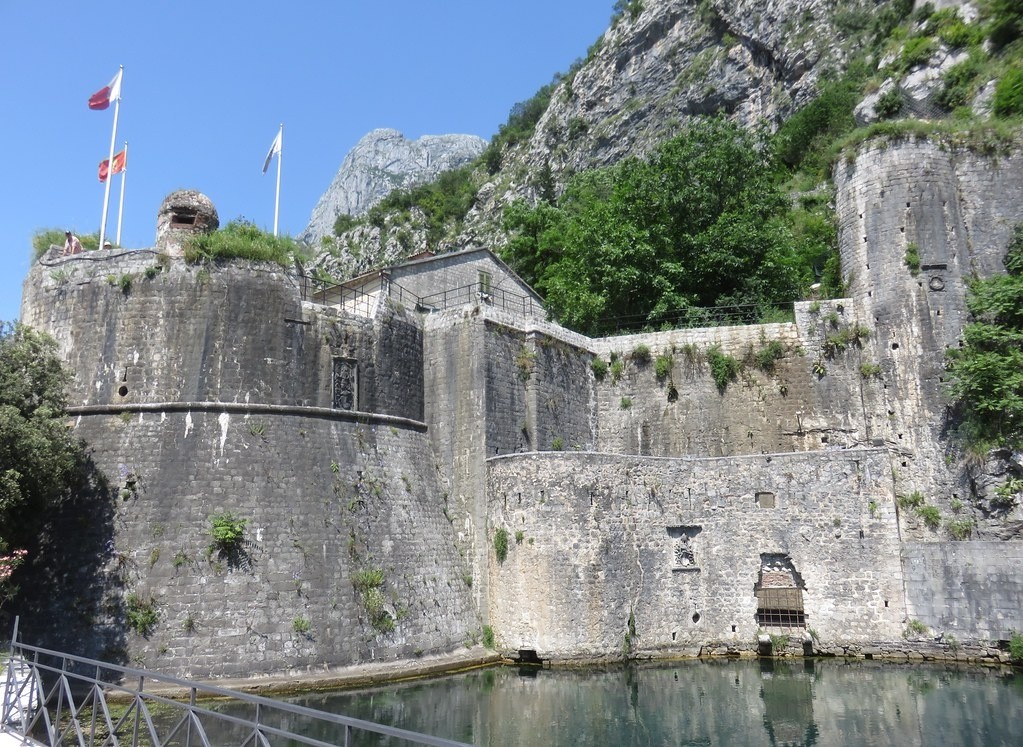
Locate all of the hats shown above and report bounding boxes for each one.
[65,231,71,235]
[104,242,111,247]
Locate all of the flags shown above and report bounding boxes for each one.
[262,129,282,175]
[88,70,122,110]
[98,148,126,184]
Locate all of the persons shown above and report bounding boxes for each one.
[104,241,112,249]
[62,230,83,256]
